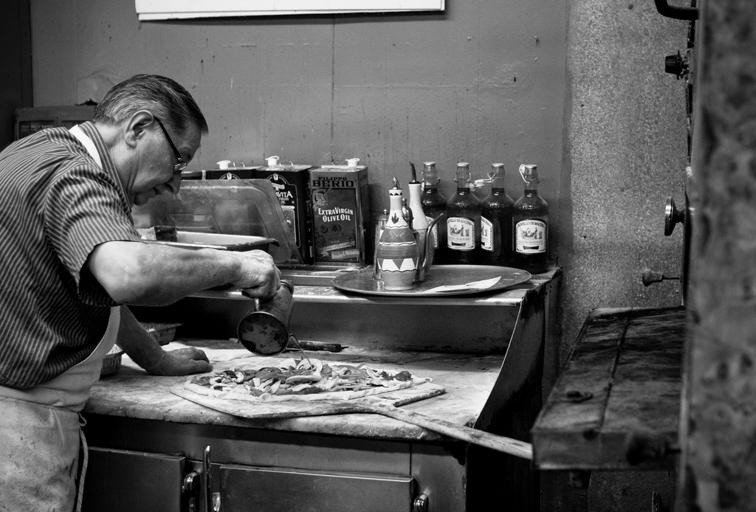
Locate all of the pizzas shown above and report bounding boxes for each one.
[183,360,434,402]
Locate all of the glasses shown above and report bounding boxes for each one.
[152,115,191,174]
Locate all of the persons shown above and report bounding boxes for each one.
[0,73,284,512]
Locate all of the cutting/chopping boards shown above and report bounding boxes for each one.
[165,353,537,462]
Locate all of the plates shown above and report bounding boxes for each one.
[330,263,534,300]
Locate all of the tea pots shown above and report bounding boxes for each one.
[233,277,304,359]
[371,157,448,292]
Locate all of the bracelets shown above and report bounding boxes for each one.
[144,348,170,375]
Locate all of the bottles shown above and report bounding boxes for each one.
[477,160,518,267]
[441,159,483,265]
[417,158,451,266]
[506,157,551,274]
